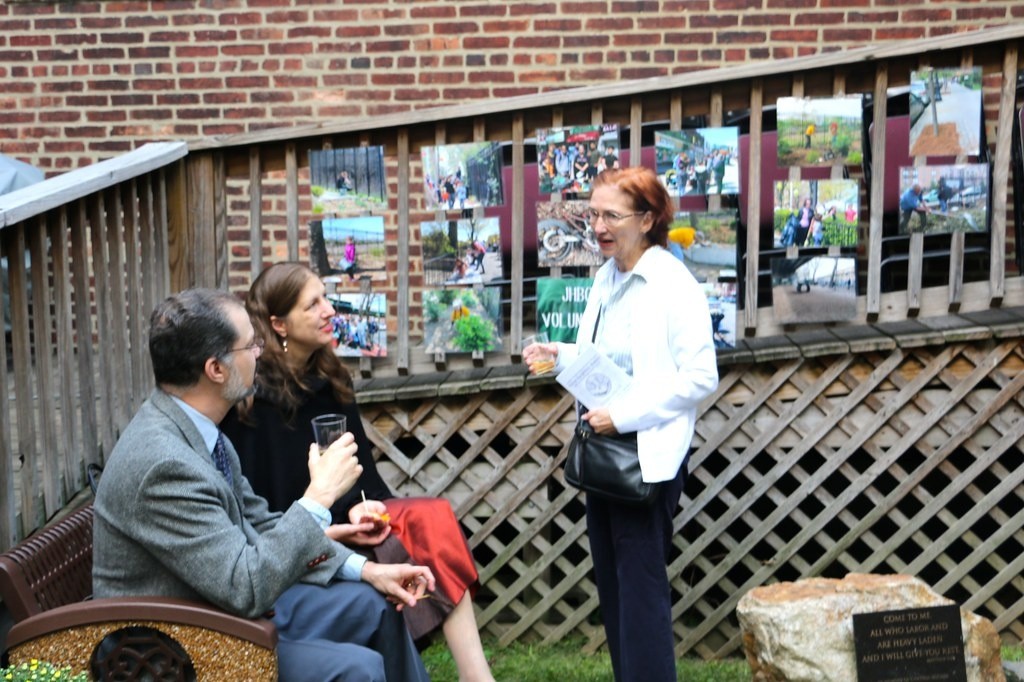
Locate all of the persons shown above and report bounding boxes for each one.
[299,69,991,362]
[88,286,440,682]
[218,258,500,682]
[518,164,720,682]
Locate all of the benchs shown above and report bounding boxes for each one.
[1,495,279,682]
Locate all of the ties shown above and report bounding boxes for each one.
[210,432,234,499]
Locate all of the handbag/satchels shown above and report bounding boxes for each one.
[564,419,659,502]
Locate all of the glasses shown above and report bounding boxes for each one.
[222,331,266,358]
[581,207,649,224]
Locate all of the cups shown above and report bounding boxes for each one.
[311,413,348,457]
[521,331,556,376]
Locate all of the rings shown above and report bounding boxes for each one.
[407,582,412,589]
